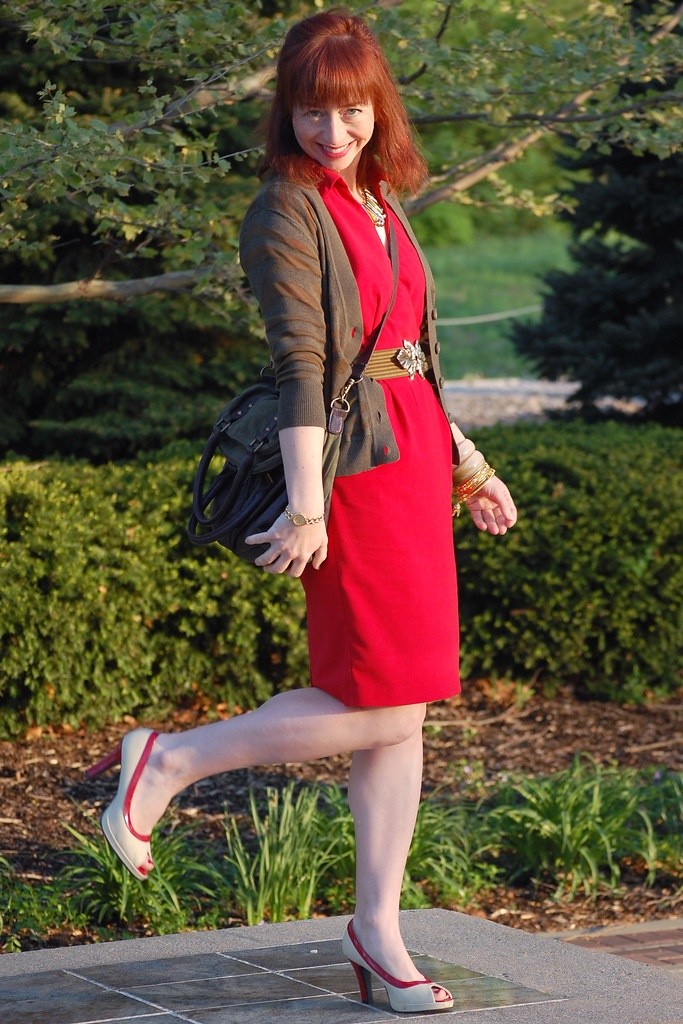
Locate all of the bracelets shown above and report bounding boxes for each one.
[452,439,496,517]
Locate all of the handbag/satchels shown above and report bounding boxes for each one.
[185,369,344,564]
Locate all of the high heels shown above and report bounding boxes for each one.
[342,918,454,1013]
[85,729,153,880]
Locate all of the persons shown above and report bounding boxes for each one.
[84,11,517,1014]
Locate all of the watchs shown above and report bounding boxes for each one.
[284,507,324,526]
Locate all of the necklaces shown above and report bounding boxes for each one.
[362,188,386,226]
